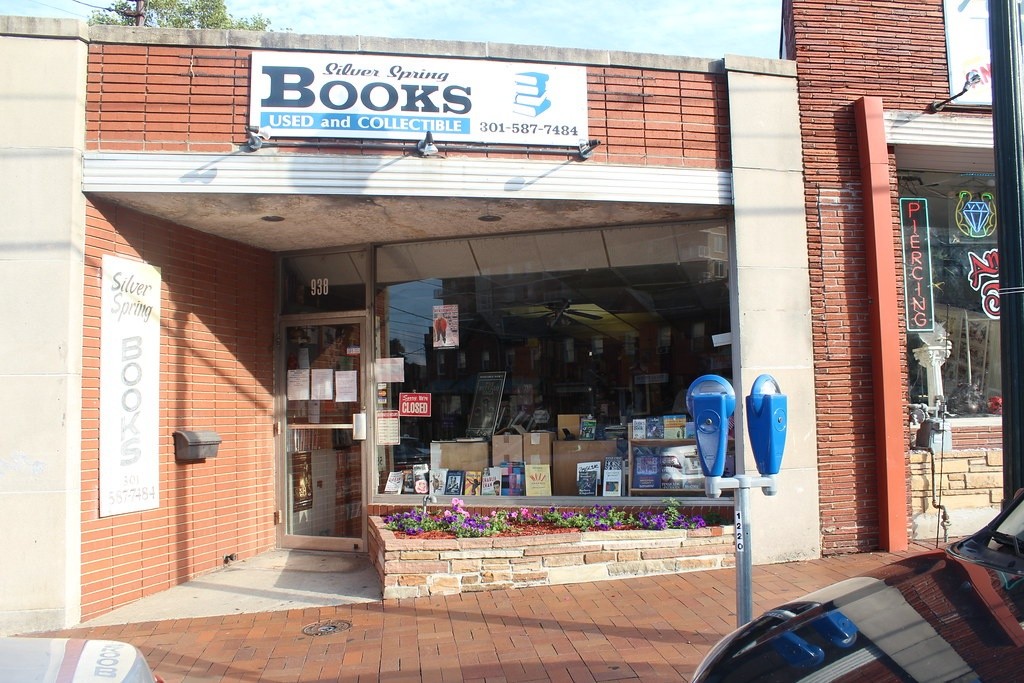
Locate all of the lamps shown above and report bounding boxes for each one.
[245,125,272,151]
[417,130,440,155]
[543,314,571,328]
[577,137,602,160]
[930,69,982,113]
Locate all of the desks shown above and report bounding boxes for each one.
[553,439,625,496]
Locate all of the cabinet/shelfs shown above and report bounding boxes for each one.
[627,422,733,497]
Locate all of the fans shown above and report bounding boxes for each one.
[529,302,603,327]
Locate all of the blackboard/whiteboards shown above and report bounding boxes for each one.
[465,371,506,436]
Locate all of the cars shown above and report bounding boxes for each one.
[0,635,165,683]
[685,483,1024,683]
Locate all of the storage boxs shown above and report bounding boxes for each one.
[429,441,493,471]
[557,414,589,440]
[522,429,557,467]
[491,424,527,467]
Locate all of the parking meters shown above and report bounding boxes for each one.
[760,601,860,671]
[685,373,788,629]
